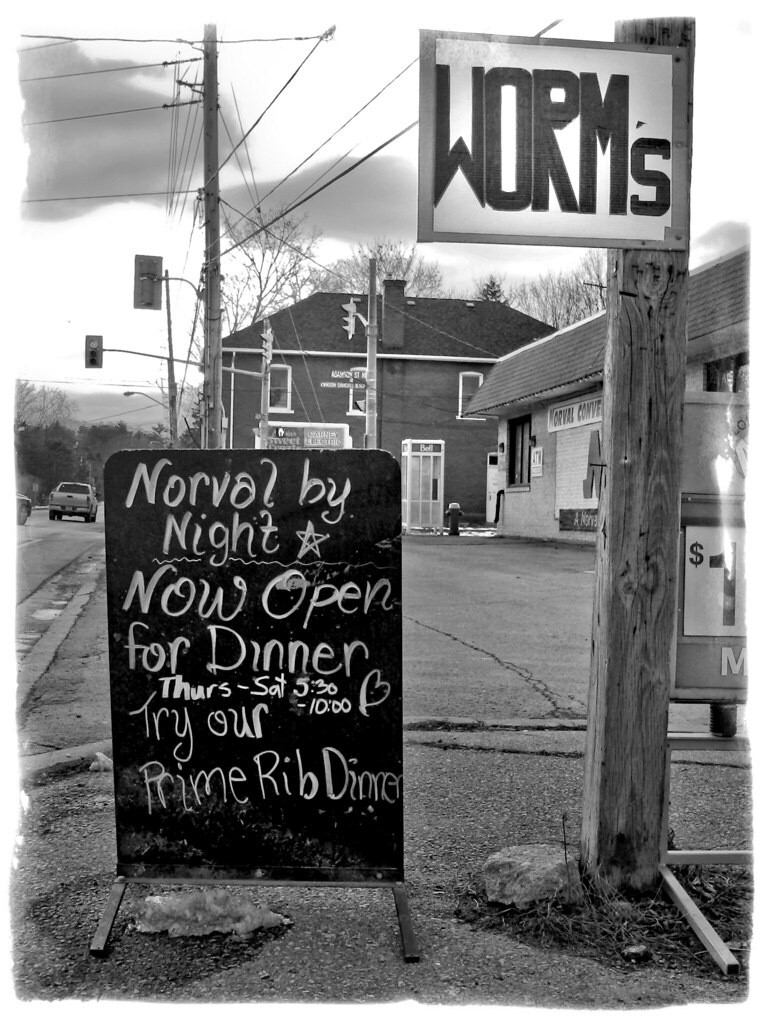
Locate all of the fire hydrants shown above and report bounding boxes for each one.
[446,503,464,537]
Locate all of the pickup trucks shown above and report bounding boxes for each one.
[48,481,102,523]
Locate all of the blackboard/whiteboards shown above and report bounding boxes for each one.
[103,448,406,886]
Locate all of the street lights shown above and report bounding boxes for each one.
[123,391,178,449]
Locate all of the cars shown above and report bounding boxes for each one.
[15,494,32,526]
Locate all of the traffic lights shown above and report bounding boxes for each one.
[85,335,102,368]
[355,400,366,412]
[260,332,274,364]
[341,303,355,340]
